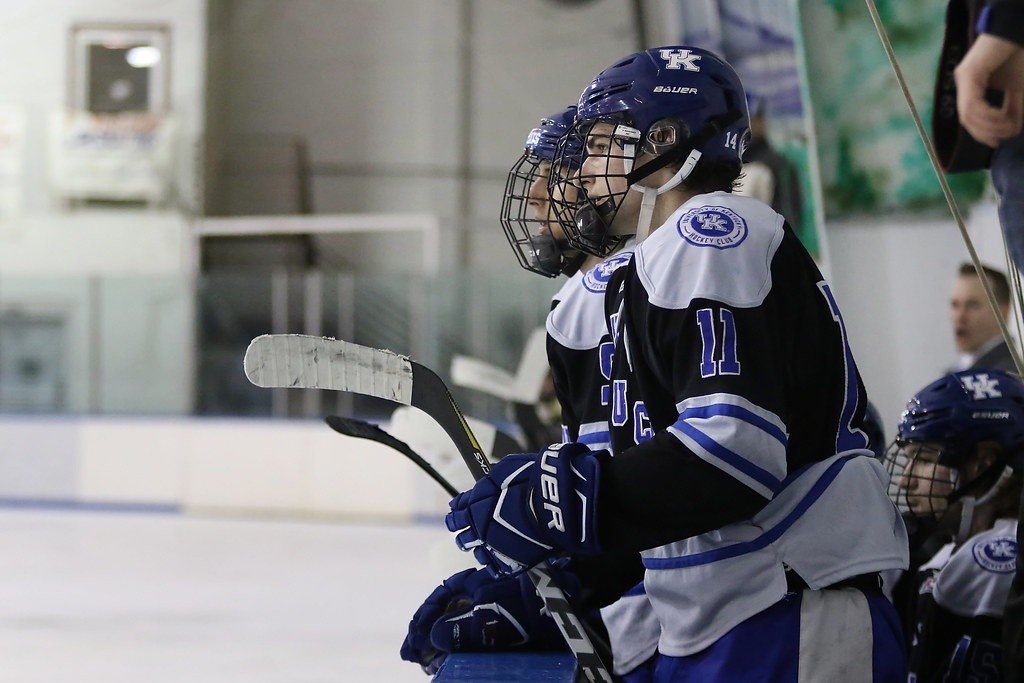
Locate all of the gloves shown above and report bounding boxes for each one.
[447,442,611,583]
[400,559,599,676]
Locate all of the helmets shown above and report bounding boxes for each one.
[547,45,752,258]
[500,103,586,278]
[885,368,1024,529]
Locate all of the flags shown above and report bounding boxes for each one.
[672,0,802,118]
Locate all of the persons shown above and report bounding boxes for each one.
[879,369,1024,683]
[930,0,1024,275]
[399,48,909,683]
[743,91,804,235]
[950,264,1017,378]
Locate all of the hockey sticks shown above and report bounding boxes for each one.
[448,351,541,406]
[388,404,524,460]
[321,412,461,501]
[241,329,617,683]
